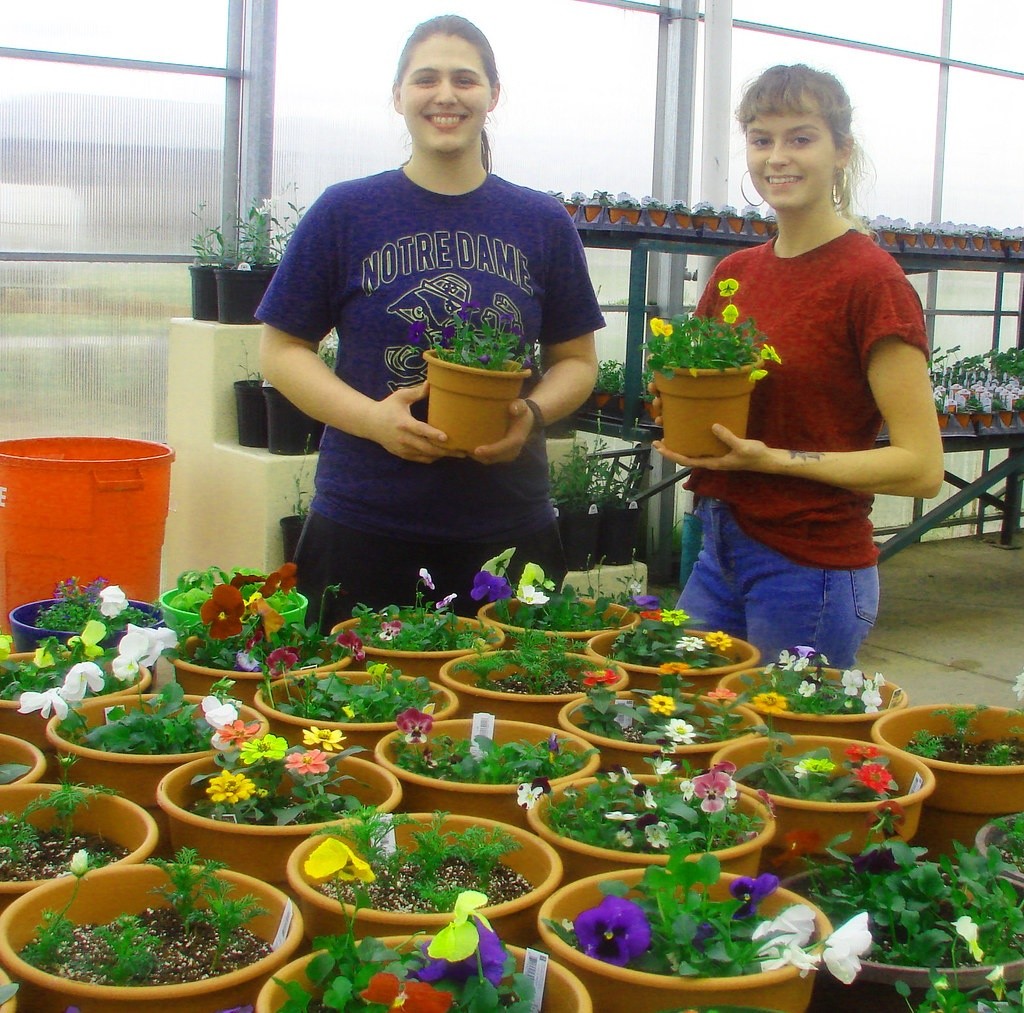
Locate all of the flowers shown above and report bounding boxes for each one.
[638,267,790,384]
[0,542,1024,1013]
[405,284,535,382]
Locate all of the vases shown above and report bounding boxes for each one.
[257,923,594,1013]
[538,858,836,1013]
[780,859,1024,1013]
[870,702,1024,863]
[165,630,356,709]
[45,692,268,843]
[719,665,911,742]
[159,581,309,650]
[284,813,566,948]
[527,772,778,884]
[556,689,771,776]
[584,625,760,697]
[422,347,532,456]
[0,732,48,785]
[653,350,765,457]
[330,611,506,684]
[374,716,605,835]
[8,597,165,651]
[474,592,642,654]
[0,784,159,915]
[158,750,400,884]
[0,648,154,784]
[974,814,1024,883]
[253,668,459,765]
[0,855,308,1013]
[711,733,937,881]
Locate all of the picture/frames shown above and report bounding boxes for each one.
[439,648,630,734]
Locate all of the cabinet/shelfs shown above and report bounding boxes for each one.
[575,223,1024,572]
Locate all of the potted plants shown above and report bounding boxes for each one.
[189,179,1024,573]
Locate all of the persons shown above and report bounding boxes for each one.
[648,63,941,667]
[255,16,607,637]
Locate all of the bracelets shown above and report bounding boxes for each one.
[525,398,544,435]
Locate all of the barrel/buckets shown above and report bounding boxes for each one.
[0,438,175,638]
